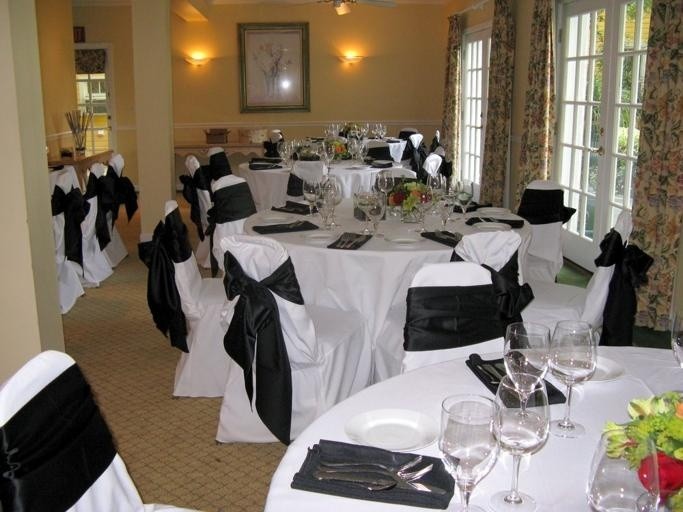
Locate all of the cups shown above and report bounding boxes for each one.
[435,193,455,239]
[314,187,333,232]
[503,321,551,432]
[426,173,447,219]
[355,192,373,235]
[376,168,395,219]
[547,319,597,441]
[323,144,336,170]
[302,137,313,148]
[358,142,367,166]
[349,123,358,140]
[340,121,349,136]
[323,123,334,137]
[585,428,662,512]
[379,125,388,141]
[455,181,473,222]
[302,176,322,222]
[487,372,553,512]
[320,175,343,228]
[437,392,503,512]
[347,139,358,166]
[365,190,387,238]
[291,137,302,161]
[412,186,434,233]
[670,311,682,373]
[333,123,340,136]
[372,122,381,140]
[277,142,289,165]
[363,120,370,140]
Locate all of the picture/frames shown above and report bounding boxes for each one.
[235,19,311,115]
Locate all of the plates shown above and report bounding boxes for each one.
[584,354,625,384]
[477,207,512,218]
[344,407,439,452]
[473,221,511,232]
[385,234,427,246]
[300,232,341,243]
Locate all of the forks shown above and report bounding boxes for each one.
[319,455,424,474]
[335,232,347,249]
[316,462,434,483]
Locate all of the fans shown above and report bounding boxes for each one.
[270,1,398,15]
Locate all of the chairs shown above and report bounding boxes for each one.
[427,128,440,154]
[201,145,233,188]
[416,153,445,189]
[515,178,577,285]
[520,208,656,345]
[108,152,142,270]
[203,173,258,279]
[136,200,225,399]
[213,234,374,446]
[399,126,420,141]
[178,153,213,242]
[405,132,430,174]
[285,160,329,207]
[447,149,457,179]
[448,229,536,371]
[48,163,90,316]
[434,144,448,185]
[81,162,116,290]
[373,167,417,196]
[0,348,201,512]
[262,128,287,159]
[377,260,507,369]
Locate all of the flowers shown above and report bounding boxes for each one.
[328,139,349,159]
[388,174,439,219]
[606,389,682,512]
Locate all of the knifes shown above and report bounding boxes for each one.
[314,470,446,496]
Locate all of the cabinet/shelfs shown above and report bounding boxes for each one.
[175,143,265,159]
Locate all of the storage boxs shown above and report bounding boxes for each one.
[203,128,232,143]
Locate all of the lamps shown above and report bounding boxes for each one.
[337,51,367,64]
[184,51,212,65]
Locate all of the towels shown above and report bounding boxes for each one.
[467,215,524,228]
[421,230,460,248]
[254,221,318,235]
[466,353,566,408]
[454,204,493,213]
[327,232,372,250]
[251,158,282,163]
[371,163,392,168]
[387,139,400,143]
[250,163,281,169]
[53,165,64,170]
[271,200,317,214]
[292,440,455,509]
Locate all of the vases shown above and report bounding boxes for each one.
[402,207,422,225]
